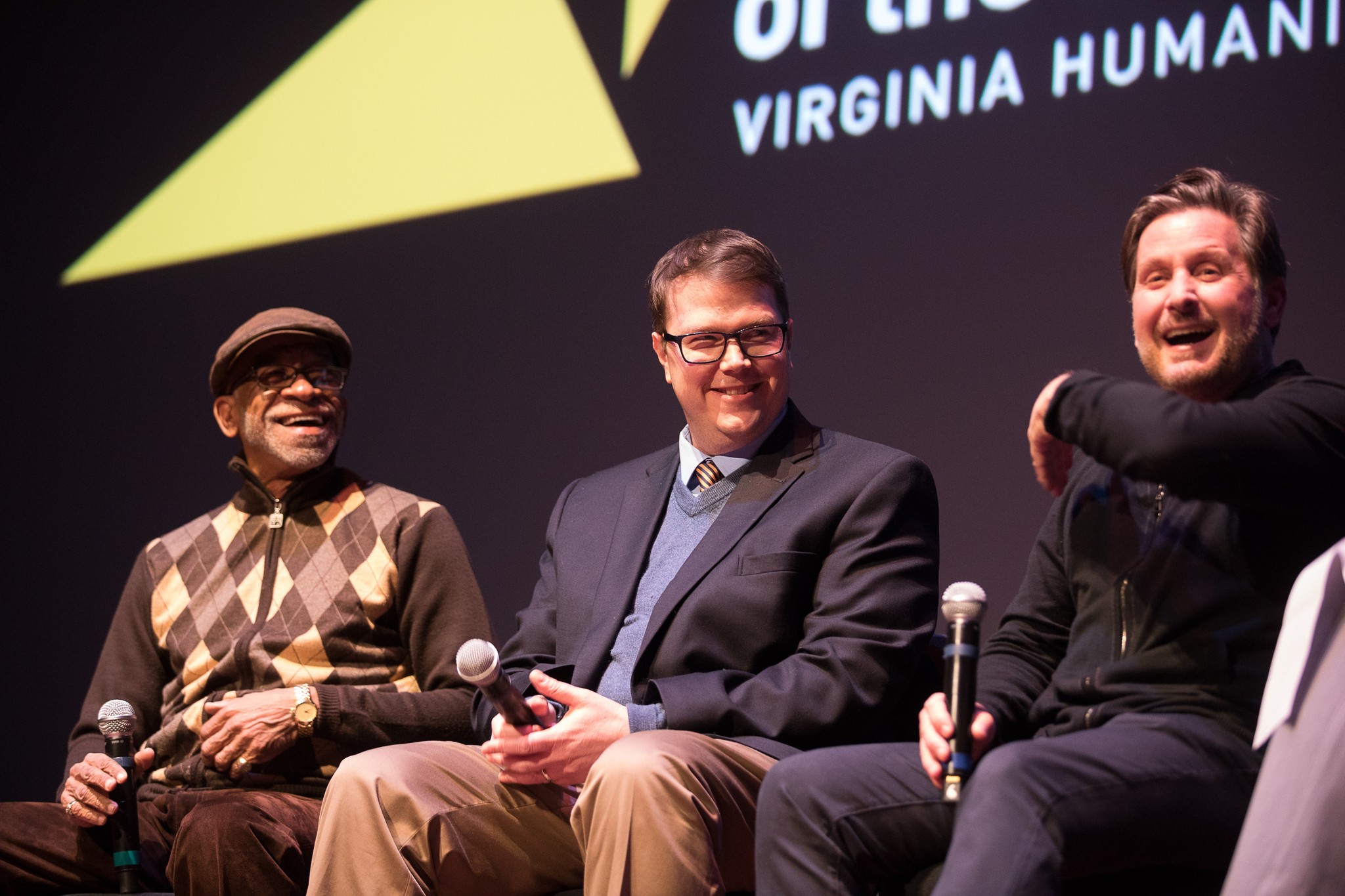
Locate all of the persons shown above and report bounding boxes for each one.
[299,228,934,896]
[0,304,496,895]
[751,163,1345,896]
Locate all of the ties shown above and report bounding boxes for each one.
[695,460,722,492]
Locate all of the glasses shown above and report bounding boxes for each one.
[661,322,788,363]
[237,364,351,391]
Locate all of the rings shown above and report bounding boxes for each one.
[64,800,76,815]
[238,756,248,773]
[541,770,552,782]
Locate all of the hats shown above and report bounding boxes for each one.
[208,308,353,397]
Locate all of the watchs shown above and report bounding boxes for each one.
[293,683,317,739]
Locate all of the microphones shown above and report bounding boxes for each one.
[937,582,983,801]
[454,639,543,738]
[98,699,140,896]
